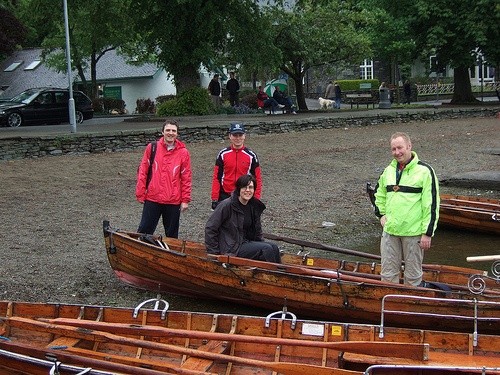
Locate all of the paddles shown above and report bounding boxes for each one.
[262,232,487,276]
[40,319,430,362]
[0,315,365,375]
[210,253,452,293]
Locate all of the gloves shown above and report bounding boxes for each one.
[212,202,217,210]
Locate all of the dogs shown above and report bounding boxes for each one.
[317,97,336,109]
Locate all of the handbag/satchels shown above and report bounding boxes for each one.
[137,166,153,189]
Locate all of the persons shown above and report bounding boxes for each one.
[334,83,340,109]
[136,119,191,238]
[325,81,336,100]
[274,86,296,114]
[210,124,263,210]
[372,132,440,286]
[403,80,411,104]
[379,82,388,103]
[208,74,220,102]
[226,72,239,106]
[204,175,281,263]
[257,86,285,115]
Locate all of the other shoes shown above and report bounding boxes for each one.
[278,104,285,109]
[291,104,296,108]
[292,111,298,115]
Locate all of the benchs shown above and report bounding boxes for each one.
[263,96,298,114]
[44,337,82,346]
[350,97,375,109]
[179,340,232,371]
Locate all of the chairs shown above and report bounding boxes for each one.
[44,93,51,103]
[39,95,43,100]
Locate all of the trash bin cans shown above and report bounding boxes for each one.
[379,88,390,108]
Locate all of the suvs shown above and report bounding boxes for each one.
[0,85,94,127]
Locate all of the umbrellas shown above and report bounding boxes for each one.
[264,78,288,98]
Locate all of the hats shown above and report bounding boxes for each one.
[229,123,245,134]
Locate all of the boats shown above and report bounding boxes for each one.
[366,181,500,239]
[100,218,500,338]
[0,292,500,375]
